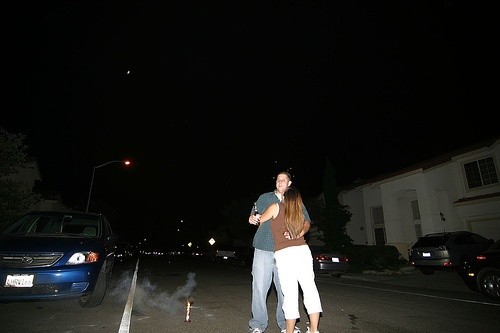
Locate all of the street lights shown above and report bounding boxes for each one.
[85,159,130,212]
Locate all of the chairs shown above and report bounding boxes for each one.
[46,220,63,234]
[82,226,96,237]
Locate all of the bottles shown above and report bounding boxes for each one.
[253,202,260,226]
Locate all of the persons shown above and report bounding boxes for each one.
[260,187,324,333]
[248,170,311,333]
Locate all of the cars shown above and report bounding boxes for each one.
[462,248,500,299]
[312,247,346,277]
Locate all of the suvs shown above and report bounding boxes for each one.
[0,212,113,308]
[407,231,500,273]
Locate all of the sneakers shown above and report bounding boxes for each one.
[281,328,301,333]
[253,328,260,333]
[304,326,319,333]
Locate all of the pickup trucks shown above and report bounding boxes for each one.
[208,247,235,264]
